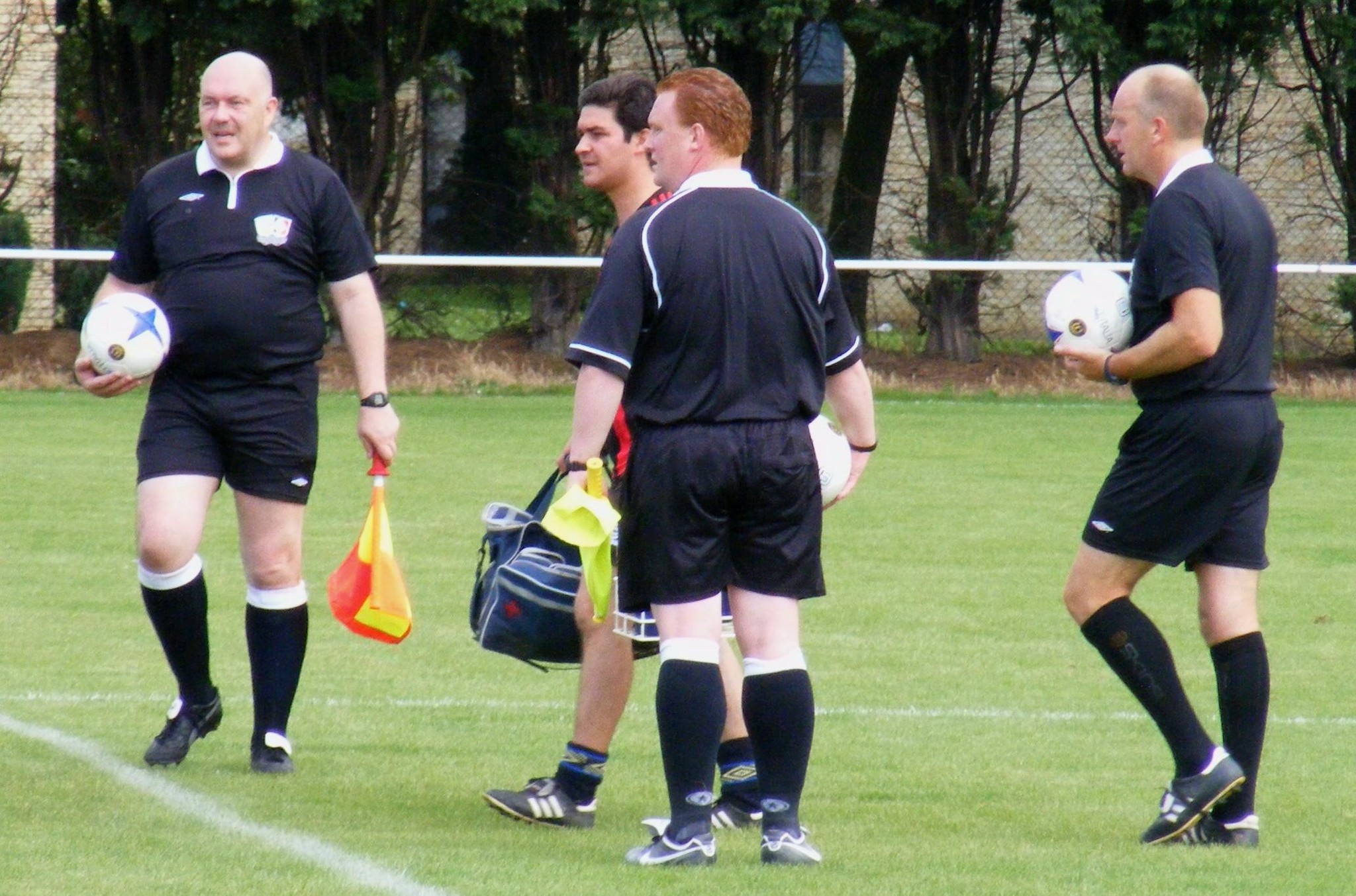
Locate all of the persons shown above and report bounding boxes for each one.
[563,69,879,868]
[1052,63,1284,848]
[74,53,402,774]
[483,73,765,839]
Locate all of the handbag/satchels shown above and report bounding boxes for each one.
[467,456,660,672]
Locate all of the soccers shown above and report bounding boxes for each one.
[1043,267,1136,363]
[809,412,854,509]
[79,291,172,382]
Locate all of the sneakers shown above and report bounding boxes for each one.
[144,685,223,768]
[622,821,717,867]
[761,825,822,866]
[1173,803,1261,852]
[250,727,293,774]
[710,803,768,829]
[483,778,595,832]
[1141,751,1247,844]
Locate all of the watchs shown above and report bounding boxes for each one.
[359,390,391,409]
[1104,354,1130,386]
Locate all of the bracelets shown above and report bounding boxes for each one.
[847,443,880,454]
[566,462,586,472]
